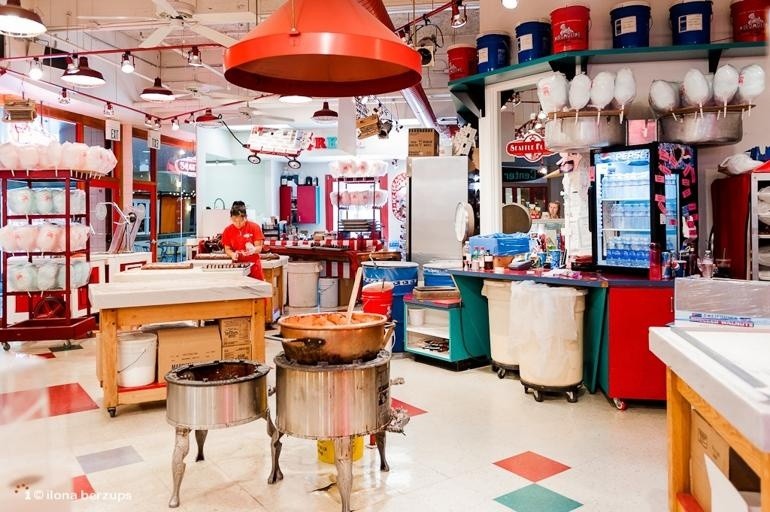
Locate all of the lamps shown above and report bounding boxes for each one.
[187,46,206,68]
[138,54,174,102]
[60,55,107,87]
[0,9,48,39]
[66,53,81,75]
[28,59,44,80]
[153,118,161,131]
[447,4,471,31]
[310,99,337,123]
[171,115,181,130]
[104,101,119,117]
[119,51,135,74]
[195,109,218,123]
[145,112,152,126]
[59,86,74,107]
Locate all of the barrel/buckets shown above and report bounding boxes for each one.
[609,1,654,55]
[477,31,514,74]
[116,334,157,387]
[549,3,594,57]
[319,277,338,307]
[360,280,395,322]
[515,19,552,65]
[726,0,769,46]
[447,43,477,84]
[668,2,714,47]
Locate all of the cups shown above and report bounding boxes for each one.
[538,252,546,267]
[549,250,561,269]
[675,261,687,277]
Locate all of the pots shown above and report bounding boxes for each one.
[265,311,396,364]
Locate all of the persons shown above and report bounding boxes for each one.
[548,200,561,218]
[221,201,267,315]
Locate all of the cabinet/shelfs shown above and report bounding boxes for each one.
[710,172,770,280]
[0,170,89,350]
[598,287,677,409]
[401,266,607,396]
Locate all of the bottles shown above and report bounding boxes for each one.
[610,204,616,229]
[473,246,479,269]
[623,204,632,229]
[601,172,675,198]
[624,237,631,267]
[630,241,637,267]
[640,203,647,229]
[616,237,623,267]
[479,247,486,270]
[485,249,492,271]
[614,204,623,229]
[645,242,650,267]
[606,236,616,267]
[637,242,645,267]
[661,252,671,279]
[633,204,640,229]
[463,240,470,267]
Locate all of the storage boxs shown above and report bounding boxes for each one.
[156,325,222,387]
[217,317,249,346]
[407,126,440,156]
[220,345,252,363]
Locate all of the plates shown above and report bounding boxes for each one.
[199,263,254,275]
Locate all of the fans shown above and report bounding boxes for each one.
[74,0,261,52]
[172,67,252,102]
